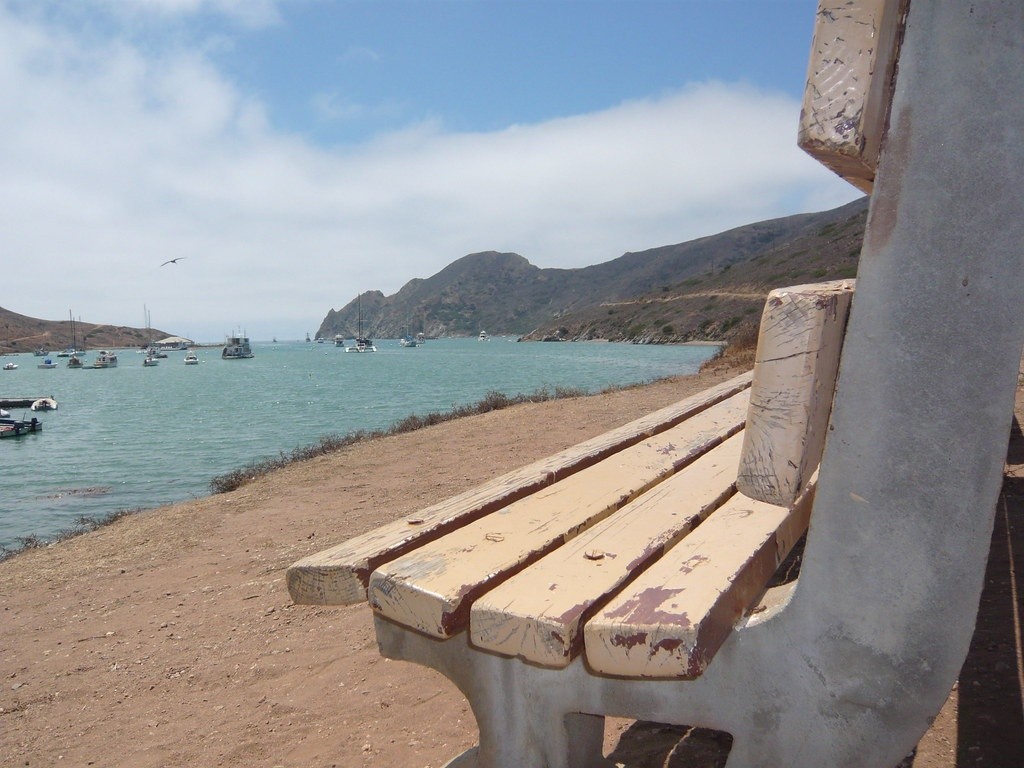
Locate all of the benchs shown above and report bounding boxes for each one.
[285,0,1024,768]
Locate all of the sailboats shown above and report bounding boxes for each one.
[57,308,86,357]
[344,292,379,353]
[399,308,426,347]
[136,303,169,366]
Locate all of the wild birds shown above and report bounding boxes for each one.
[160,256,188,267]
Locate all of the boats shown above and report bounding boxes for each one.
[184,349,200,365]
[222,325,255,359]
[478,329,490,341]
[333,333,345,347]
[306,332,311,342]
[317,337,324,343]
[1,363,19,371]
[37,358,59,369]
[30,396,59,411]
[92,350,118,369]
[33,347,49,357]
